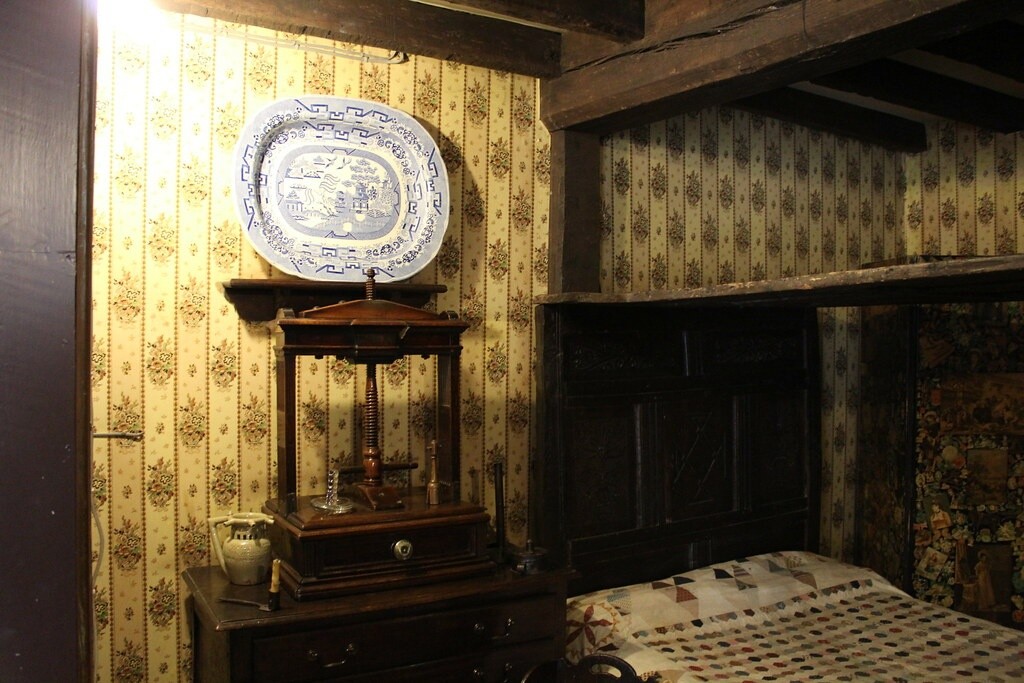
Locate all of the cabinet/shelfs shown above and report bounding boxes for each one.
[181,562,569,683]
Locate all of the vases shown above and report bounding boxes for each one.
[207,512,275,585]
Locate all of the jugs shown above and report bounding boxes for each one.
[208,512,276,585]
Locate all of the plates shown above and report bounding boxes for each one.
[232,95,450,284]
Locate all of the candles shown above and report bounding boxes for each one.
[271,559,280,591]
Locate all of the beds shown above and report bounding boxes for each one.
[567,552,1024,683]
